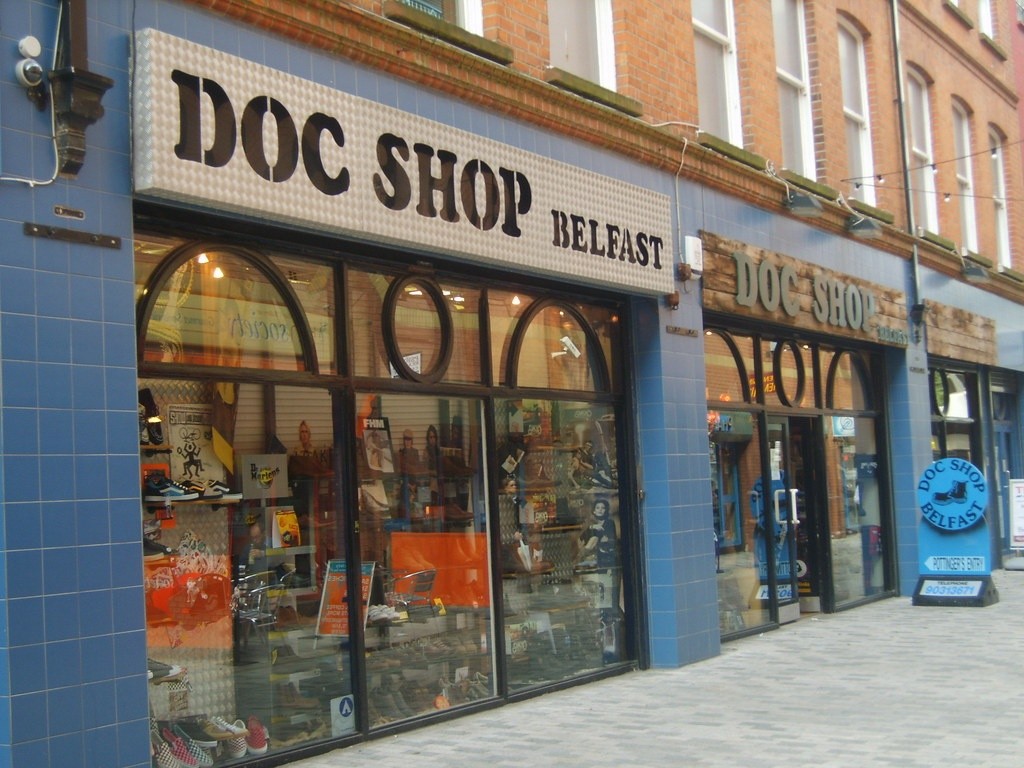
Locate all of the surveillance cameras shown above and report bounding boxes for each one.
[15,58,43,88]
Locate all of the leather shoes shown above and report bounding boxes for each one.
[288,455,335,478]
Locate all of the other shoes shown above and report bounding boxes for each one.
[139,388,163,445]
[439,457,474,476]
[395,455,430,477]
[271,613,614,746]
[358,462,382,478]
[429,498,474,521]
[268,563,311,588]
[390,506,425,522]
[138,402,149,444]
[144,538,163,561]
[151,714,267,767]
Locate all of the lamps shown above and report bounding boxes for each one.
[781,185,823,220]
[962,257,991,285]
[845,206,882,238]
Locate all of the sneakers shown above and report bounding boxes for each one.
[203,479,243,500]
[368,604,401,624]
[178,480,223,499]
[144,475,199,502]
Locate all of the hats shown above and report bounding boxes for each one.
[403,429,413,439]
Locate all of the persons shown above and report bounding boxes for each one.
[397,429,423,473]
[423,426,443,504]
[292,420,318,457]
[566,441,598,490]
[241,515,286,590]
[719,417,732,431]
[572,500,620,568]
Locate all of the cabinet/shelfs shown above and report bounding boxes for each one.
[138,394,632,768]
